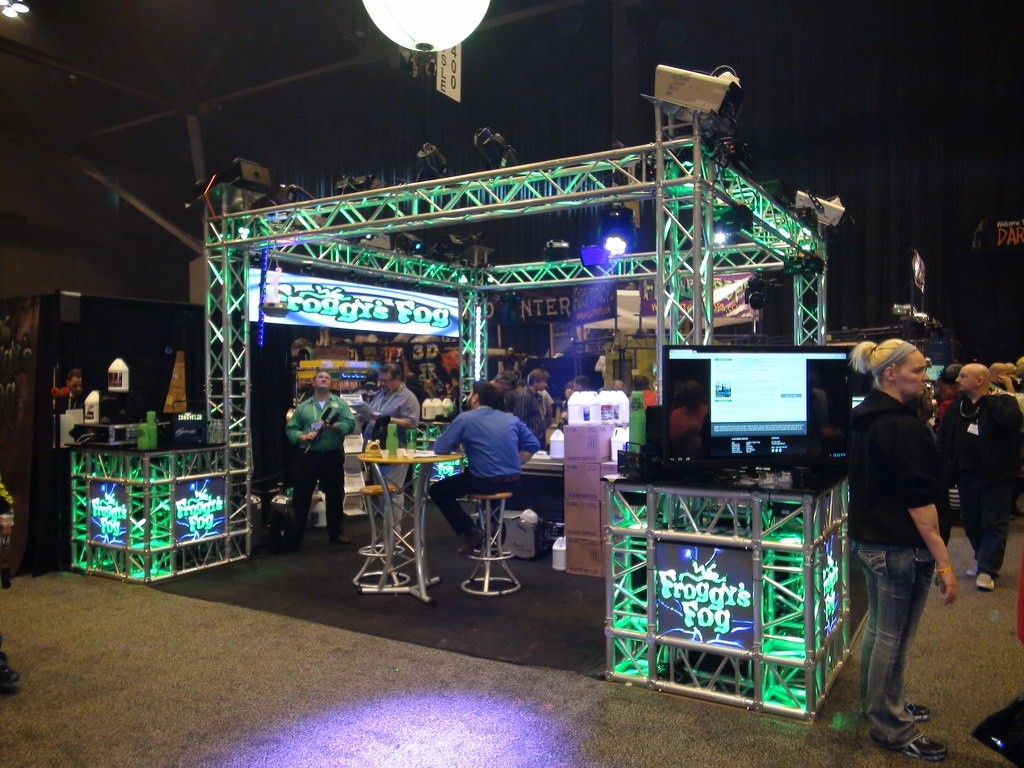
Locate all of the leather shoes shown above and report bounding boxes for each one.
[330,535,351,544]
[457,528,487,556]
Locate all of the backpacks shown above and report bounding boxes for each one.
[268,507,297,554]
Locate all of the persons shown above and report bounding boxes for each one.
[844,338,960,763]
[284,367,656,556]
[61,368,83,414]
[668,381,710,457]
[929,355,1024,589]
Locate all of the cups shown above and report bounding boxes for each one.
[630,390,647,454]
[386,424,398,459]
[407,449,414,459]
[137,411,157,450]
[406,428,416,453]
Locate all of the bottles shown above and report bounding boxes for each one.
[549,429,565,459]
[568,390,630,460]
[422,398,453,420]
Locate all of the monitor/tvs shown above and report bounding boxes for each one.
[662,344,853,497]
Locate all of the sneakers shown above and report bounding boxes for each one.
[894,735,947,762]
[976,573,994,590]
[966,566,978,576]
[903,702,930,721]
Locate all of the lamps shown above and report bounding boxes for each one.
[745,278,771,309]
[711,221,731,249]
[599,207,637,259]
[473,127,531,170]
[414,142,448,182]
[363,0,492,52]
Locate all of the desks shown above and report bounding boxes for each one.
[354,448,465,606]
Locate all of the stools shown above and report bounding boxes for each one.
[352,485,410,587]
[460,492,521,595]
